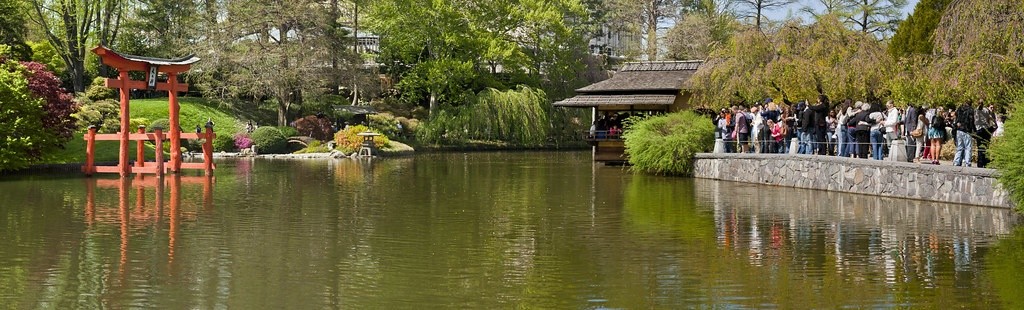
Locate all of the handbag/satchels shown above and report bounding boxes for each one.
[880,127,886,134]
[912,129,923,137]
[934,116,945,130]
[988,117,998,132]
[951,123,957,138]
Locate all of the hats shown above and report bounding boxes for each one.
[754,102,762,106]
[764,98,773,104]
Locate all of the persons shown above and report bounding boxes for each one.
[589,111,630,139]
[245,120,259,133]
[707,93,1017,168]
[316,109,326,119]
[204,117,215,133]
[289,118,296,128]
[196,123,201,140]
[396,121,404,138]
